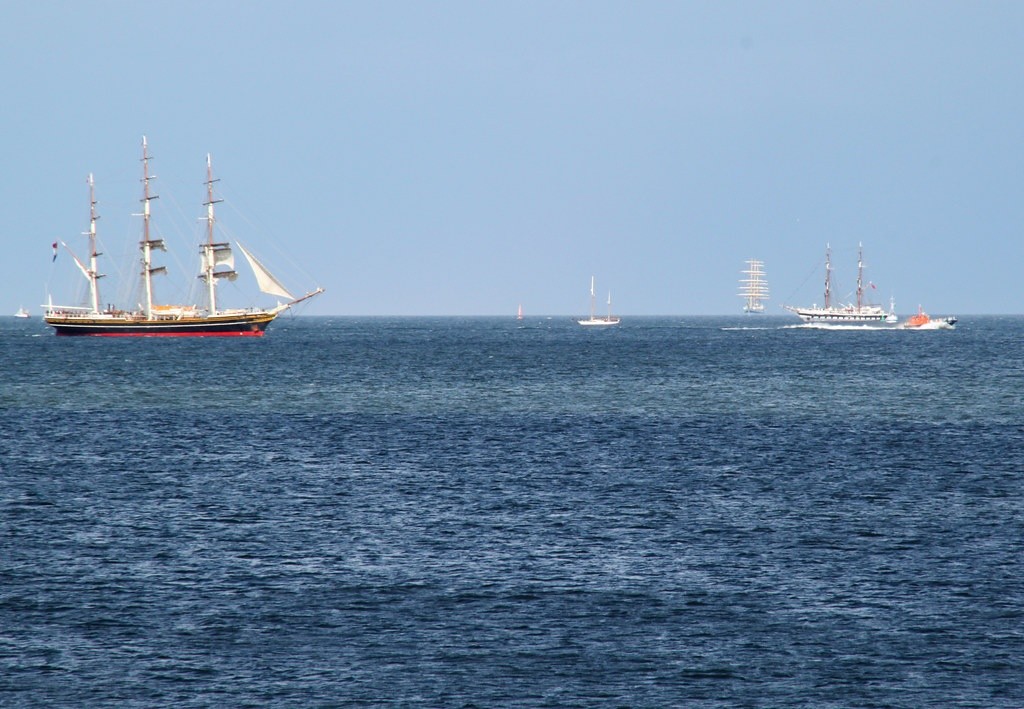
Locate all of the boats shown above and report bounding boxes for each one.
[897,302,959,328]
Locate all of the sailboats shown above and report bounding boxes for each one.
[736,256,772,314]
[38,135,325,338]
[576,274,621,326]
[780,240,889,323]
